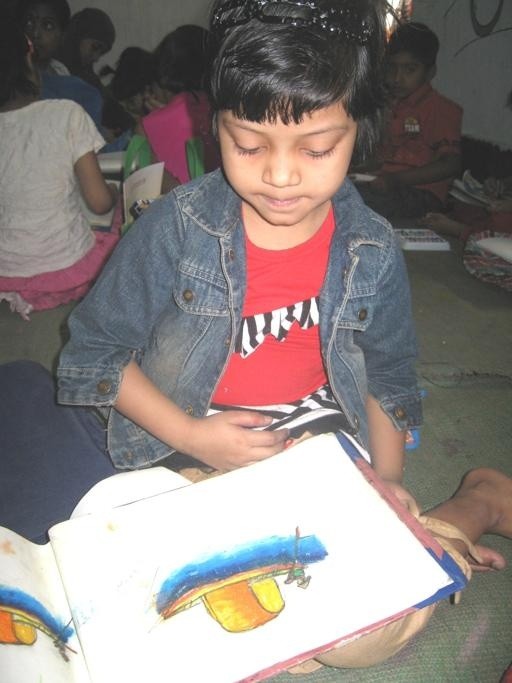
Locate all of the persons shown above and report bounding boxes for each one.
[1,1,219,310]
[56,1,511,676]
[343,21,463,220]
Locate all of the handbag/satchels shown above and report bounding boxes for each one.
[122,134,204,235]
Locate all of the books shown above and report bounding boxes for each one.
[76,180,121,233]
